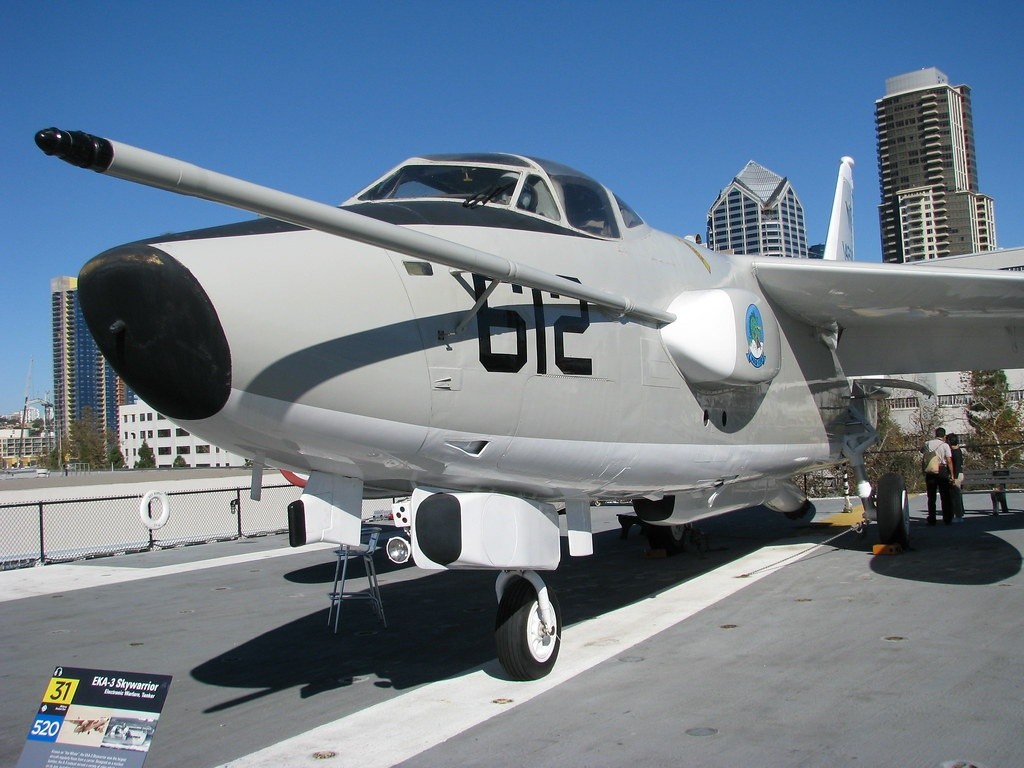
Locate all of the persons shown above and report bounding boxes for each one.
[922,427,965,524]
[63,460,69,476]
[496,172,537,212]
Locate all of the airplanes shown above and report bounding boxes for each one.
[30,125,1023,679]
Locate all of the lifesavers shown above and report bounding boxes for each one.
[141,491,172,530]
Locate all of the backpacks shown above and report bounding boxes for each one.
[924,441,944,474]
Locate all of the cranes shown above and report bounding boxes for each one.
[24,354,53,412]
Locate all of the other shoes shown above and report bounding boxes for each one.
[927,516,936,526]
[952,517,963,522]
[942,519,952,525]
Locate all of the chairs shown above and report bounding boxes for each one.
[494,172,532,211]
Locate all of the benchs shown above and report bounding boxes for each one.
[962,469,1024,515]
